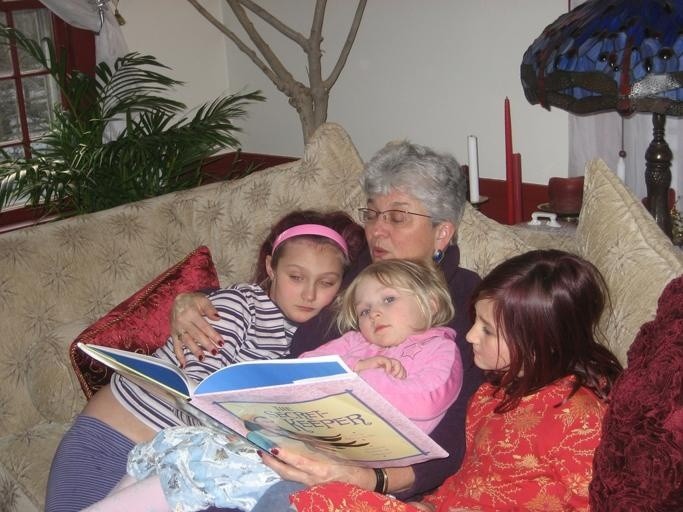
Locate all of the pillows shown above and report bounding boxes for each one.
[582,156,683,372]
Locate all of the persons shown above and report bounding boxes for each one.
[289,249,624,512]
[80,257,464,512]
[170,140,483,512]
[45,209,365,512]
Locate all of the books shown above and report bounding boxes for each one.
[78,344,450,470]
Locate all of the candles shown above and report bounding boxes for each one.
[468,135,480,203]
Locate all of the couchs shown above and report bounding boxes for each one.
[0,123,683,512]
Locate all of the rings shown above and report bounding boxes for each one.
[177,330,190,338]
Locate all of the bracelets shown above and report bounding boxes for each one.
[372,466,384,494]
[382,470,389,493]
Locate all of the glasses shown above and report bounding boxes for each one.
[357,208,430,225]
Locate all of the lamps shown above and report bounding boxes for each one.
[520,1,683,241]
